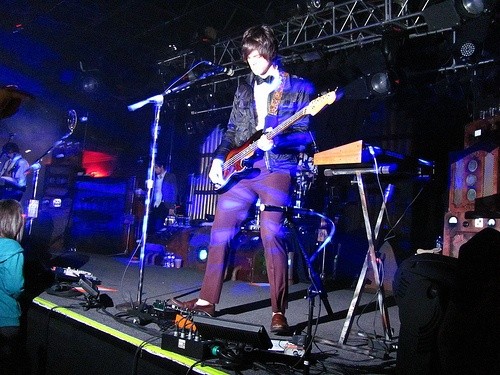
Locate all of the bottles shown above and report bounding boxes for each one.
[435,235,442,254]
[163,252,175,268]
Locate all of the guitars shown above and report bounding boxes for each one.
[212,84,342,195]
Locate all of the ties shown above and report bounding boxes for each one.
[254,75,275,84]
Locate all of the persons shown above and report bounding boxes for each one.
[0,142,30,202]
[171,25,317,332]
[0,199,32,375]
[128,157,177,264]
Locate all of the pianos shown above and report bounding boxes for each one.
[314,140,435,176]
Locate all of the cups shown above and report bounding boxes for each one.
[174,259,182,268]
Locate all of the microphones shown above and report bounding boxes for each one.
[260,204,314,215]
[208,61,234,76]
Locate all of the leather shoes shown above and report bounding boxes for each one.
[171,297,215,316]
[270,314,289,333]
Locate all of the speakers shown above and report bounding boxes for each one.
[442,148,500,259]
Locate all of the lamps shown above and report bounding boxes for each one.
[422,0,487,31]
[368,27,401,99]
